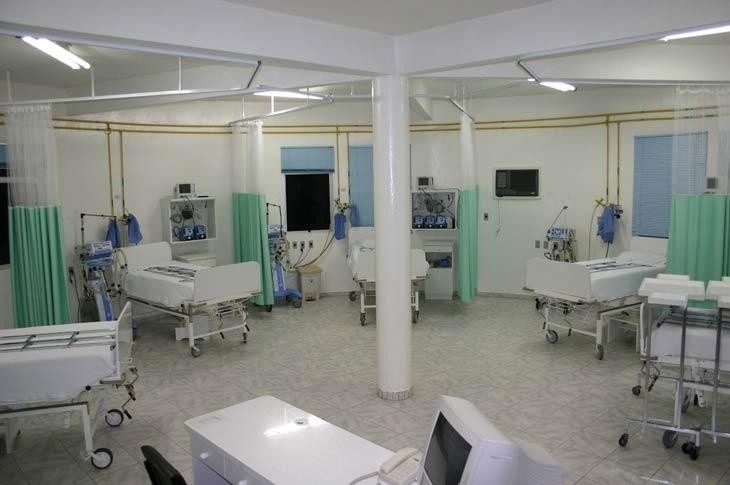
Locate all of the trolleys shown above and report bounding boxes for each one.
[617,274,729,460]
[265,202,304,312]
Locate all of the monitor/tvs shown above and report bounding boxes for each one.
[420,396,561,484]
[493,168,540,199]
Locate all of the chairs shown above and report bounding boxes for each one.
[140,446,188,485]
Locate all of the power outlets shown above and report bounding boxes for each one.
[292,240,313,249]
[534,240,559,251]
[483,214,488,219]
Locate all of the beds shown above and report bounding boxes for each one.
[116,241,262,357]
[0,300,138,468]
[524,251,667,359]
[347,226,429,326]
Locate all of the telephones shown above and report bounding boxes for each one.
[378,447,425,485]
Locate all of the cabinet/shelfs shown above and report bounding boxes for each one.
[185,396,417,485]
[175,252,217,266]
[161,198,217,244]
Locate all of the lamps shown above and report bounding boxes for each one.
[528,79,577,92]
[254,91,324,100]
[659,25,730,41]
[21,35,91,70]
